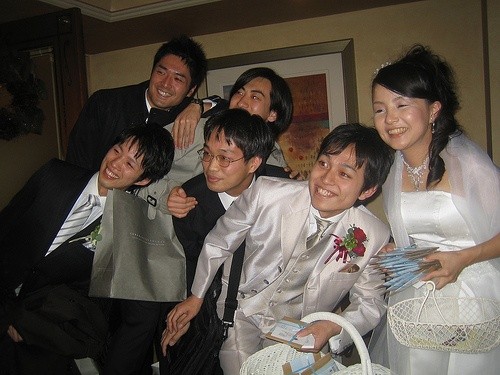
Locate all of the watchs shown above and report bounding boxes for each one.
[191,99,205,113]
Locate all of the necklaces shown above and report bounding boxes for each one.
[401,154,430,192]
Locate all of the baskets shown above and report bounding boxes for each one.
[387,281,500,353]
[239,341,347,375]
[330,361,391,375]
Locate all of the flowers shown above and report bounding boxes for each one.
[325,223,368,265]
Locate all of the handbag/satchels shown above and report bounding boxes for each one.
[159,294,224,375]
[88,188,187,302]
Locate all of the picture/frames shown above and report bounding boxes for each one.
[197,38,360,181]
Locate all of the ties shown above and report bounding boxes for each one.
[306,212,333,249]
[44,194,101,258]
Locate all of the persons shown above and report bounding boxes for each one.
[133,69,303,218]
[0,123,175,375]
[368,46,500,375]
[168,123,395,375]
[172,108,297,375]
[66,36,231,173]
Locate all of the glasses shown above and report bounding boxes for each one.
[197,147,248,168]
